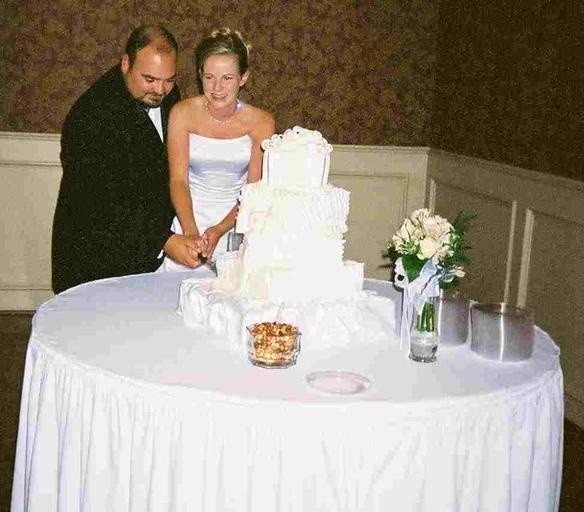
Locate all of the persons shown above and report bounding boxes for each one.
[153,25,275,275]
[46,23,205,299]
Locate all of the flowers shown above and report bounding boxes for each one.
[385,208,477,332]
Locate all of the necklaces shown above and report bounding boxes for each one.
[203,97,242,126]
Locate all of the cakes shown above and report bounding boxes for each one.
[209,126,365,303]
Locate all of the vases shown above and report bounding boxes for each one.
[409,289,443,363]
